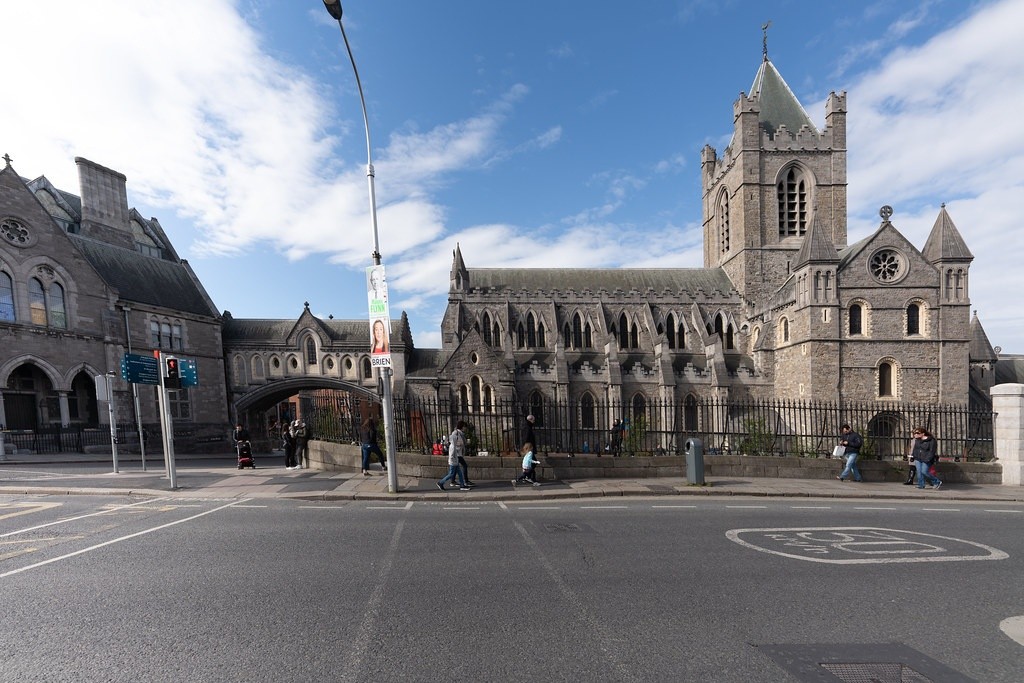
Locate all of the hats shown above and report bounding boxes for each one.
[294,420,301,426]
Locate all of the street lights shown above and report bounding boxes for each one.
[322,0,398,492]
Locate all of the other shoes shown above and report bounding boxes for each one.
[533,482,541,487]
[450,481,461,487]
[511,479,516,488]
[465,481,475,487]
[854,480,862,483]
[933,481,942,490]
[523,477,533,483]
[285,465,303,470]
[382,466,387,471]
[364,472,372,477]
[436,482,446,491]
[836,475,843,482]
[460,486,471,491]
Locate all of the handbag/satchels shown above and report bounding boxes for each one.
[833,444,846,456]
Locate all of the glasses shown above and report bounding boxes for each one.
[915,432,920,434]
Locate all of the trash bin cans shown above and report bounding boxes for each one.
[685,437,704,484]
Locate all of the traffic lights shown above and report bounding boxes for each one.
[166,358,181,389]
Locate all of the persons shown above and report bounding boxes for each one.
[609,419,623,457]
[137,426,148,455]
[269,420,283,451]
[511,443,541,488]
[371,320,388,353]
[431,438,443,455]
[282,419,308,469]
[360,417,387,476]
[519,415,537,484]
[368,269,387,306]
[911,428,943,489]
[903,428,916,485]
[836,424,863,482]
[232,423,251,469]
[436,420,475,491]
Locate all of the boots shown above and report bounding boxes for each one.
[903,470,915,485]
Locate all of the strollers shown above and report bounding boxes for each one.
[237,441,256,470]
